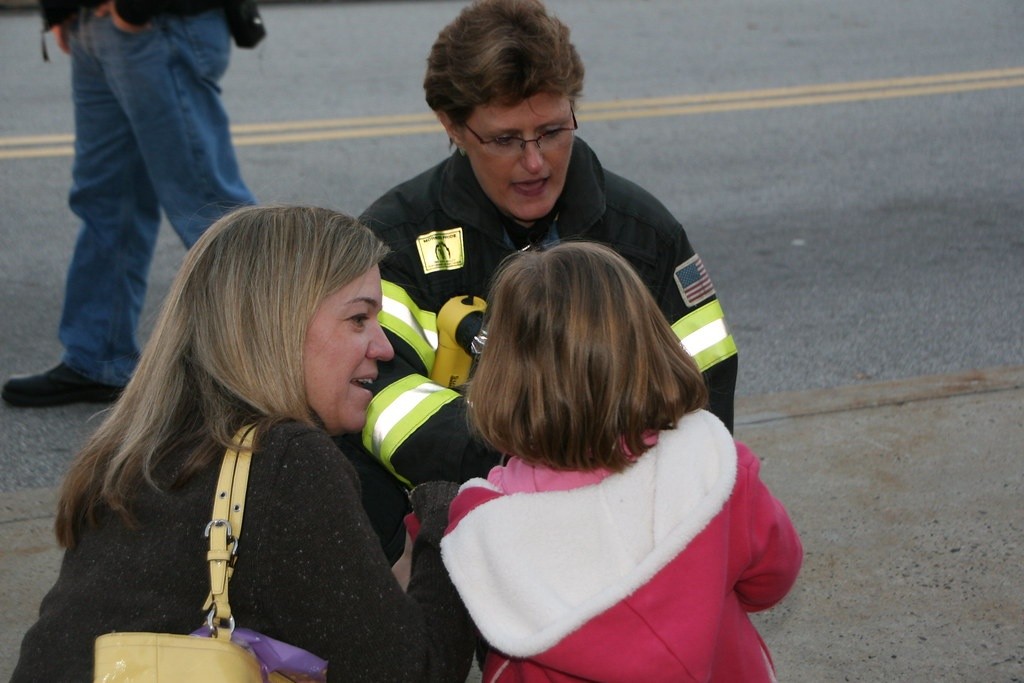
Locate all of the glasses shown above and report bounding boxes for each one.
[458,107,578,157]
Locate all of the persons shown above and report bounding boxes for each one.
[0,0,269,410]
[17,204,476,683]
[334,1,740,566]
[403,244,803,683]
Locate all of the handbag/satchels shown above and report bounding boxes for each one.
[95,422,330,683]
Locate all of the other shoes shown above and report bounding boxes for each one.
[1,361,124,407]
